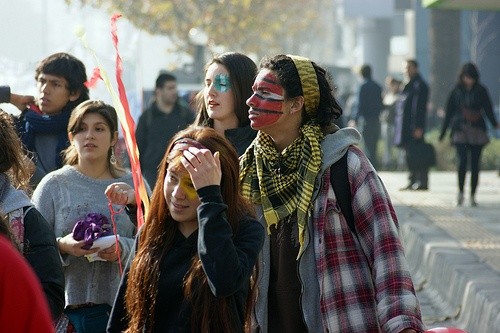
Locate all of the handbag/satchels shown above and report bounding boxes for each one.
[407,139,435,170]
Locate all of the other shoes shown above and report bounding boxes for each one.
[416,187,427,190]
[400,187,411,190]
[471,195,475,206]
[458,193,463,206]
